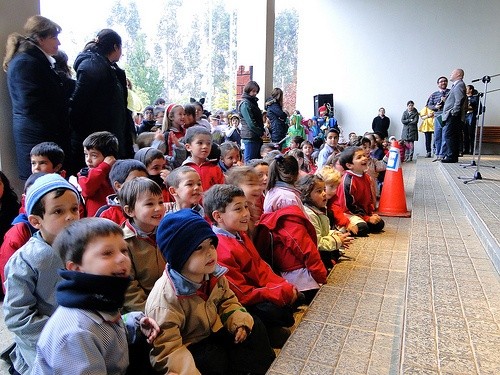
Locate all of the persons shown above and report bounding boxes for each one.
[372,108,390,140]
[4,15,137,181]
[146,208,275,375]
[401,100,419,162]
[428,77,451,162]
[203,184,298,348]
[0,124,385,311]
[0,170,80,375]
[140,81,402,208]
[32,218,160,375]
[438,69,466,163]
[463,85,479,155]
[419,98,435,157]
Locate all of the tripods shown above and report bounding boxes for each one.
[457,73,500,184]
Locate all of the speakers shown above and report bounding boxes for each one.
[313,94,334,118]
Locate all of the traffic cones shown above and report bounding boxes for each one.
[375,141,412,219]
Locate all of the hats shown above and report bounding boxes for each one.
[24,173,80,219]
[156,207,218,273]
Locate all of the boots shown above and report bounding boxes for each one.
[425,152,431,158]
[404,154,409,161]
[406,156,412,162]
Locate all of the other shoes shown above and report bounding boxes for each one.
[432,157,440,162]
[441,156,458,163]
[438,157,444,160]
